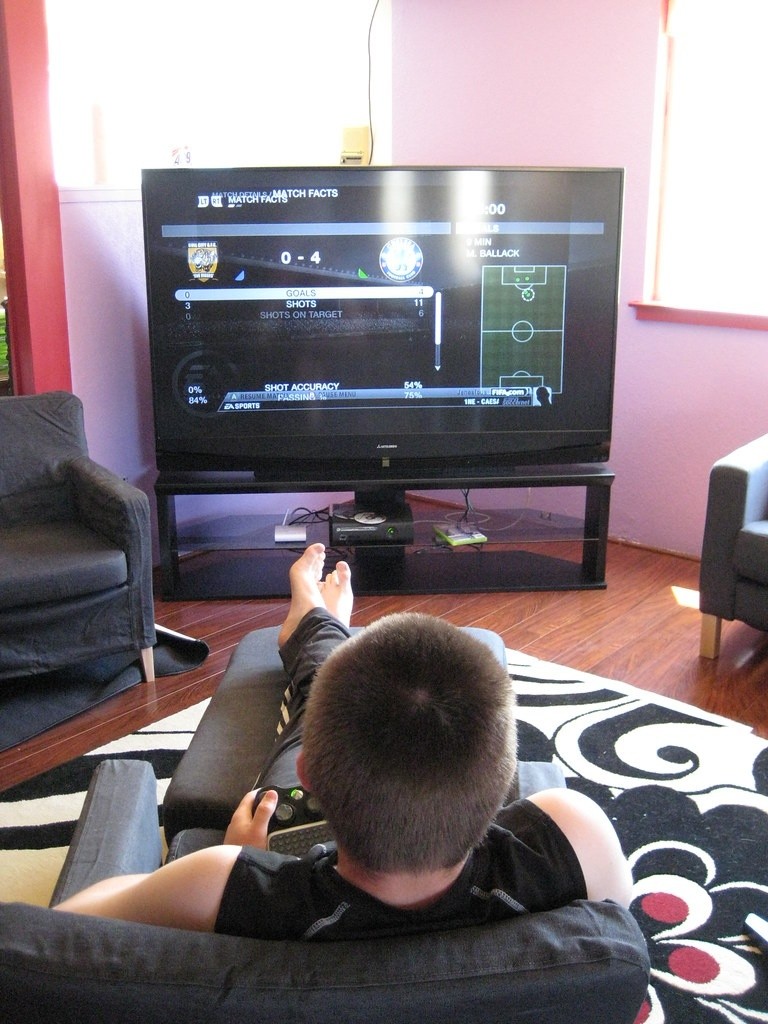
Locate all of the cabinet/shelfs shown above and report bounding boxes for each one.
[152,463,616,602]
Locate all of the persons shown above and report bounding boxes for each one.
[52,542,635,940]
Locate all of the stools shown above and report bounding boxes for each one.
[161,625,509,849]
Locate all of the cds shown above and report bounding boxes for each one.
[354,513,386,524]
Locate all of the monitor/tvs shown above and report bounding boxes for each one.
[140,166,624,473]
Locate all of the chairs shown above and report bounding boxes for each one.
[697,434,768,661]
[0,392,158,684]
[0,760,651,1024]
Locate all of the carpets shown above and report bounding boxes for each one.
[0,647,768,1024]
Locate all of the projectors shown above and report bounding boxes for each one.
[329,503,414,546]
